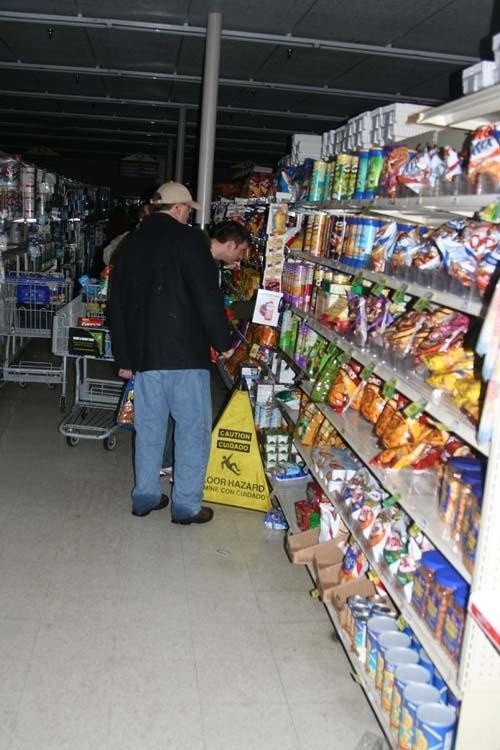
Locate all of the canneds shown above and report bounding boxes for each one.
[345,594,391,653]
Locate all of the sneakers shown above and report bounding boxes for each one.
[171,506,214,526]
[132,493,169,517]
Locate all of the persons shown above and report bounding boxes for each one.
[103,200,150,265]
[131,219,253,486]
[100,179,233,526]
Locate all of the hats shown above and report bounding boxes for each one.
[150,180,202,210]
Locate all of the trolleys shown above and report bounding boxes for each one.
[0,270,75,411]
[51,277,137,452]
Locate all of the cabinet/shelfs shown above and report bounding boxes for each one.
[0,149,112,384]
